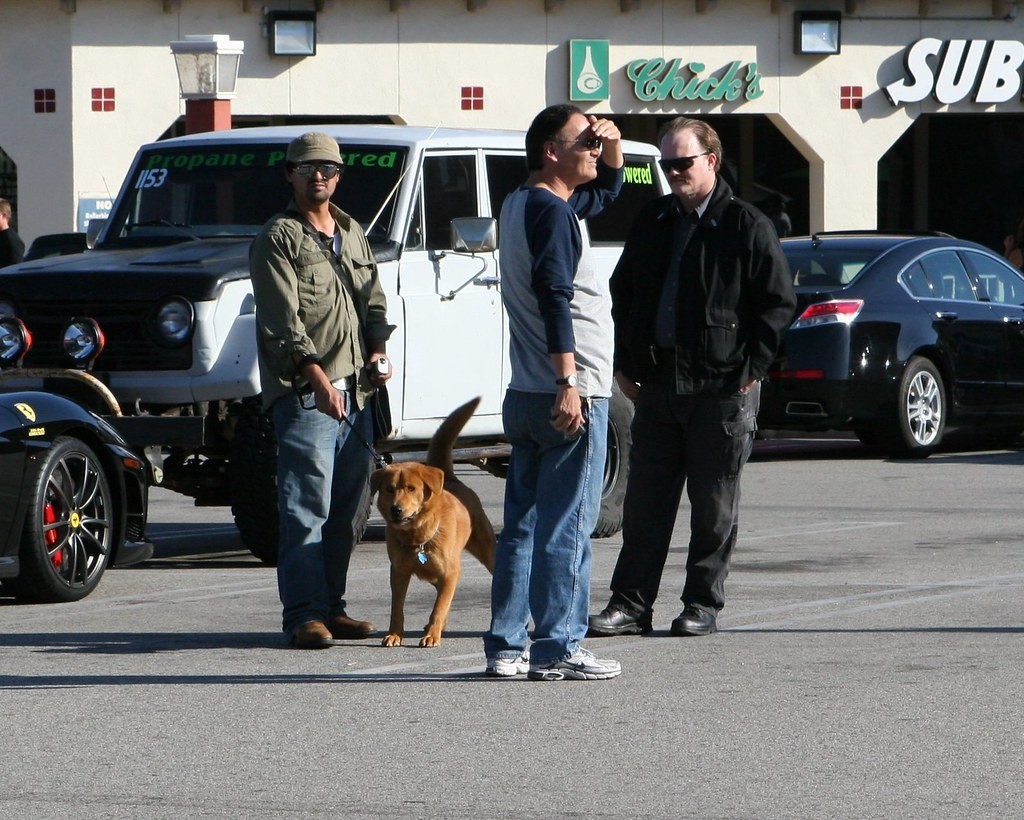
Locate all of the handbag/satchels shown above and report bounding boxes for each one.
[371,384,393,440]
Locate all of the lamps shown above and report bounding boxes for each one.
[168,32,246,101]
[793,9,842,55]
[268,8,317,57]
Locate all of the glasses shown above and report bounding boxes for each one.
[555,137,602,150]
[291,165,341,181]
[658,152,709,174]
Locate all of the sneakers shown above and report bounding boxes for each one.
[484,649,530,676]
[527,646,622,681]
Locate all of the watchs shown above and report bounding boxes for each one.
[556,374,579,387]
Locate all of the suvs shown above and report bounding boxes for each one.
[0,124,673,541]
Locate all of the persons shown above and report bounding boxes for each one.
[0,197,26,269]
[586,115,796,639]
[247,130,399,650]
[476,102,626,685]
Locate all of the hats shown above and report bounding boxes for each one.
[286,131,344,165]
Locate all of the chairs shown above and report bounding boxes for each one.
[798,257,843,286]
[428,170,474,247]
[911,267,946,299]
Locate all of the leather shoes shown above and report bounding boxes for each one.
[290,620,336,647]
[325,614,379,638]
[588,604,654,635]
[671,603,717,635]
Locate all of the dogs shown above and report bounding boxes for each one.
[368,397,496,648]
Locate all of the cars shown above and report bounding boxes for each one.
[757,228,1024,464]
[0,374,151,603]
